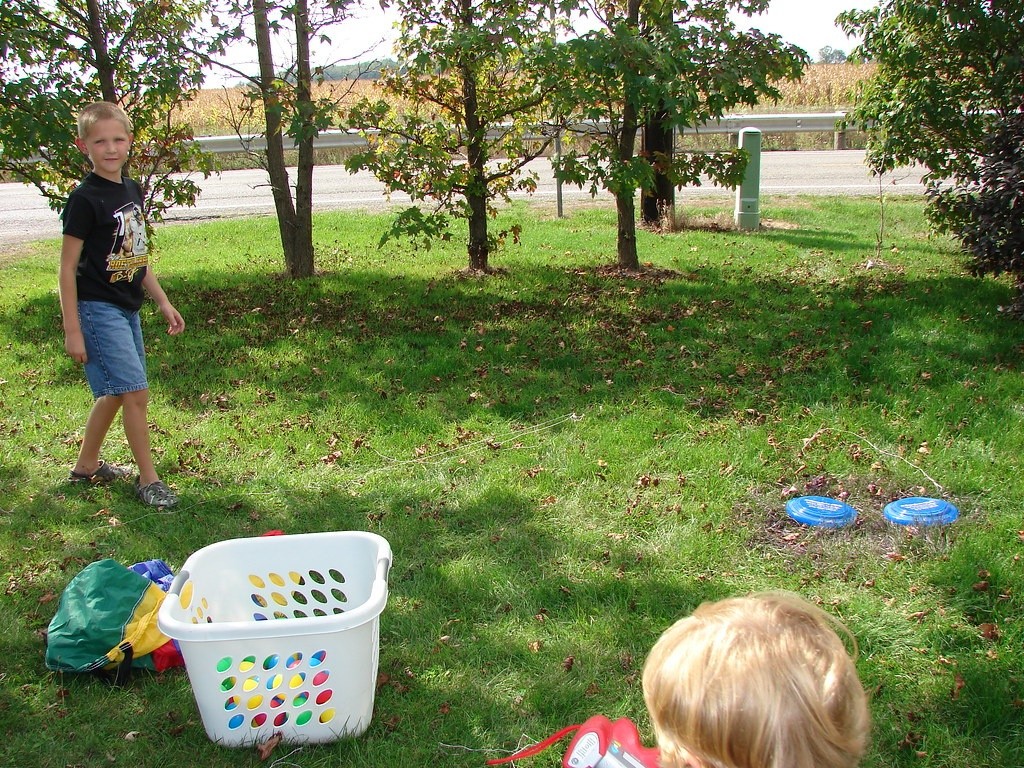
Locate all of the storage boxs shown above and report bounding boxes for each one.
[158,531,393,747]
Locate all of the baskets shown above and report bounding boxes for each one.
[157,530,392,747]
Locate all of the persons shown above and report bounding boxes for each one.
[642,592,869,767]
[60,103,185,507]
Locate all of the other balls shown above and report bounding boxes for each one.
[215,651,337,729]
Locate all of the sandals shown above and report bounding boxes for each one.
[68,460,125,483]
[133,474,180,508]
